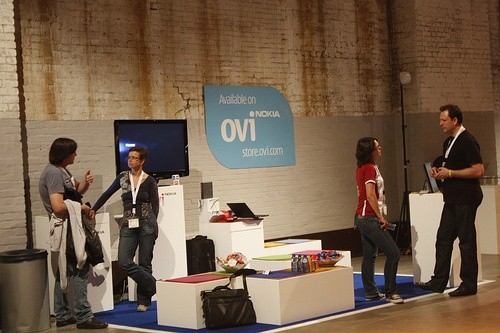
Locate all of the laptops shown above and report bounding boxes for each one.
[226,202,269,218]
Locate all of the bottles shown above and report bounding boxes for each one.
[291,254,317,273]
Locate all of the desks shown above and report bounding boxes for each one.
[209,221,265,270]
[410,184,500,286]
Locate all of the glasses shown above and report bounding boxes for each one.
[127,156,141,160]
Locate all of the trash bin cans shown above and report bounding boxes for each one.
[0,249,51,333]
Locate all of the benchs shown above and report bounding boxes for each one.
[265,238,322,257]
[242,264,356,326]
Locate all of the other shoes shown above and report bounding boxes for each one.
[448,290,464,297]
[417,281,433,289]
[386,294,404,303]
[56,317,76,327]
[137,305,148,311]
[77,317,108,329]
[365,293,385,301]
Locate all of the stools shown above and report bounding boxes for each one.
[292,249,351,268]
[156,274,232,330]
[252,256,293,272]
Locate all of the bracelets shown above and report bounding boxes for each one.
[449,170,453,178]
[379,217,385,220]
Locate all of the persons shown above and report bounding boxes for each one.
[413,105,484,296]
[354,137,403,303]
[93,146,162,311]
[39,138,107,329]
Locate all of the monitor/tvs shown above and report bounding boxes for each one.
[424,162,439,192]
[113,119,189,181]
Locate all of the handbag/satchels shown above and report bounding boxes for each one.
[200,269,257,329]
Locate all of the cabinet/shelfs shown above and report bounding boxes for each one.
[35,213,114,316]
[129,184,187,301]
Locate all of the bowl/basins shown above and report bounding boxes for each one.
[318,254,343,267]
[221,263,248,273]
